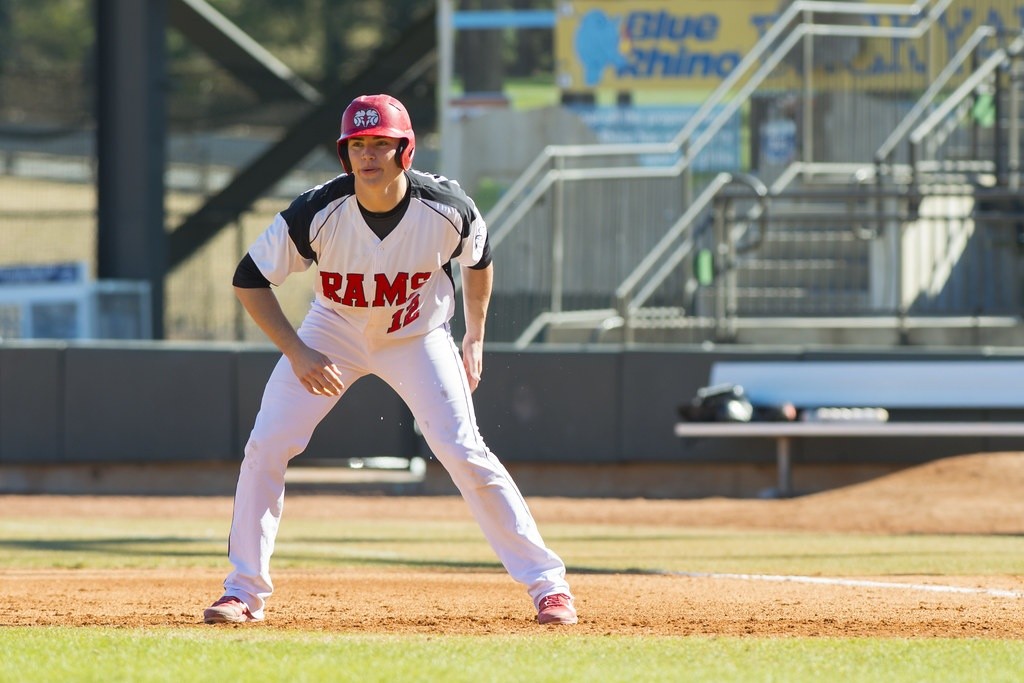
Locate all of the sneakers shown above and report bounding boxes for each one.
[536,591,579,625]
[204,595,260,623]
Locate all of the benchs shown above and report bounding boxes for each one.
[674,359,1024,499]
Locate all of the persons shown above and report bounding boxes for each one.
[205,92,580,624]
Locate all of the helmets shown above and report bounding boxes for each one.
[337,94,415,172]
